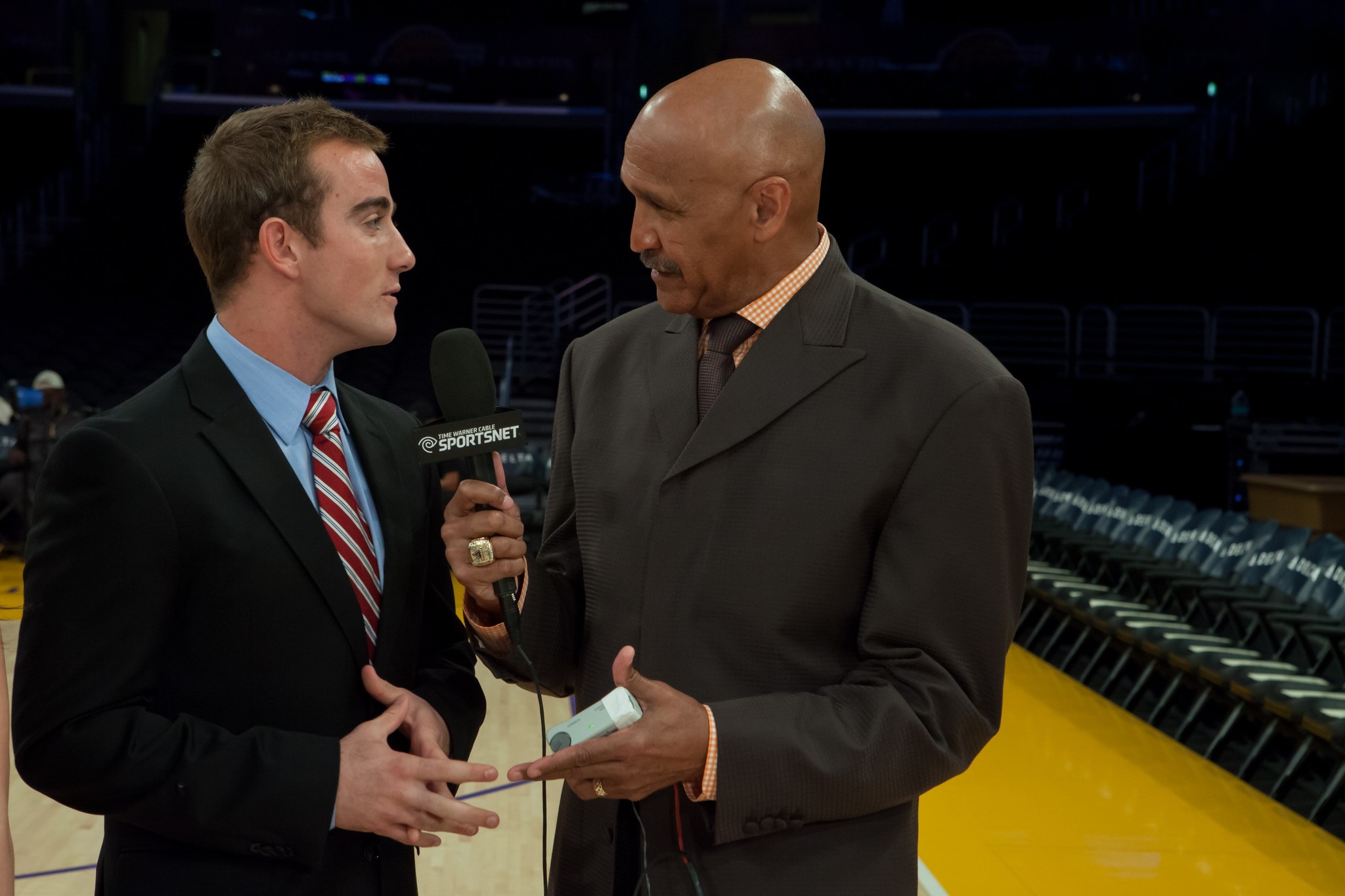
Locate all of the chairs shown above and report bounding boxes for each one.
[1012,460,1344,840]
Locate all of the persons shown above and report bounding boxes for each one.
[435,55,1034,896]
[9,91,503,896]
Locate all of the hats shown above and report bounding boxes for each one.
[30,369,64,393]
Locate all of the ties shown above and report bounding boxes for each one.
[697,313,758,434]
[304,388,379,665]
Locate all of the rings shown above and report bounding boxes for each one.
[468,538,494,568]
[592,776,608,798]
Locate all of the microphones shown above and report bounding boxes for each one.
[413,326,528,645]
